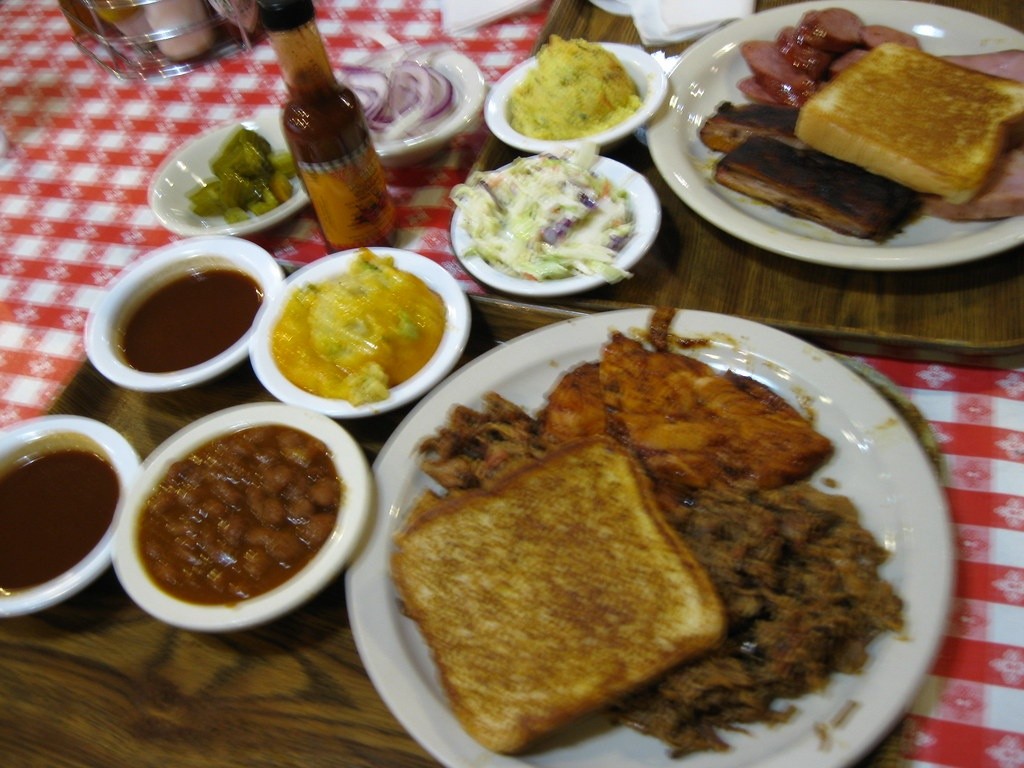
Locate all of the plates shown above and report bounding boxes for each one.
[341,302,955,768]
[82,235,288,387]
[0,415,139,619]
[146,108,311,237]
[249,247,470,416]
[449,156,662,293]
[111,403,376,633]
[482,38,669,152]
[651,1,1023,273]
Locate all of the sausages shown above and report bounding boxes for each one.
[738,7,924,110]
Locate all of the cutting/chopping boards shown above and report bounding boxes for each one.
[448,0,1022,365]
[0,267,626,767]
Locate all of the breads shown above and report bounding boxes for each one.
[793,42,1024,206]
[386,433,731,760]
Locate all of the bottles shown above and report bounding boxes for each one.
[247,0,399,254]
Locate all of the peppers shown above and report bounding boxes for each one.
[190,128,294,224]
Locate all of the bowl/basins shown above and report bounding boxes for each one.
[336,35,486,170]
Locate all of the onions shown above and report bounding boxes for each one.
[334,40,456,141]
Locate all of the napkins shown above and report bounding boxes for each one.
[442,0,545,33]
[589,0,758,46]
